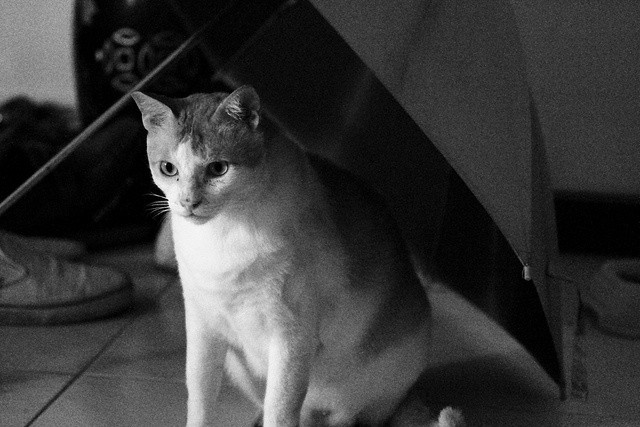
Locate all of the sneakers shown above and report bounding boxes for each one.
[1,235,92,264]
[0,233,137,324]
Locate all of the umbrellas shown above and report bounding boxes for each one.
[0,0,568,403]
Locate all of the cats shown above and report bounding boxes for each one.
[129,83,465,420]
